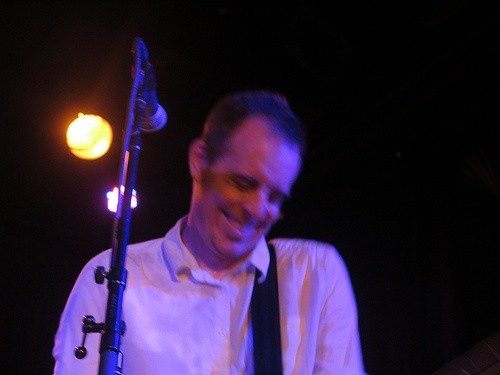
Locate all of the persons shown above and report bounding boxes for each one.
[51,93,366,375]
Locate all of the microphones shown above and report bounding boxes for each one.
[131,41,167,132]
